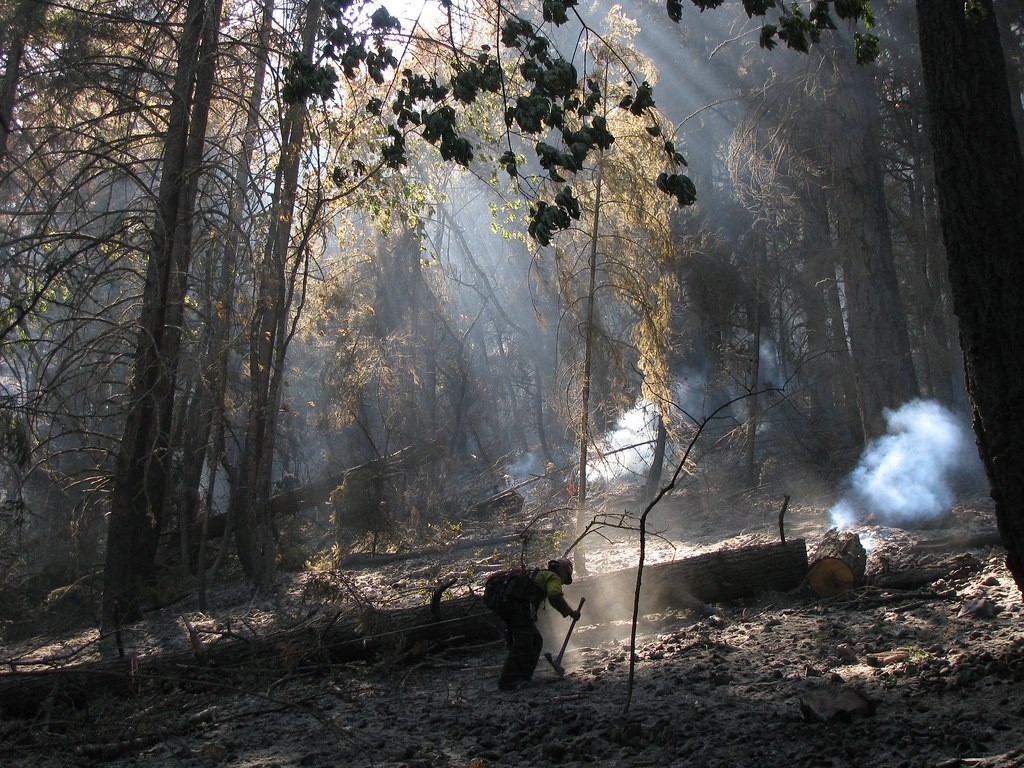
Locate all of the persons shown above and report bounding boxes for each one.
[497,557,580,690]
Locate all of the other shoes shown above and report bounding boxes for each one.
[499,679,530,689]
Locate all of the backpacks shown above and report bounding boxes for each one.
[484,567,559,632]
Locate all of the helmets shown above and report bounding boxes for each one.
[546,462,556,469]
[548,558,573,586]
